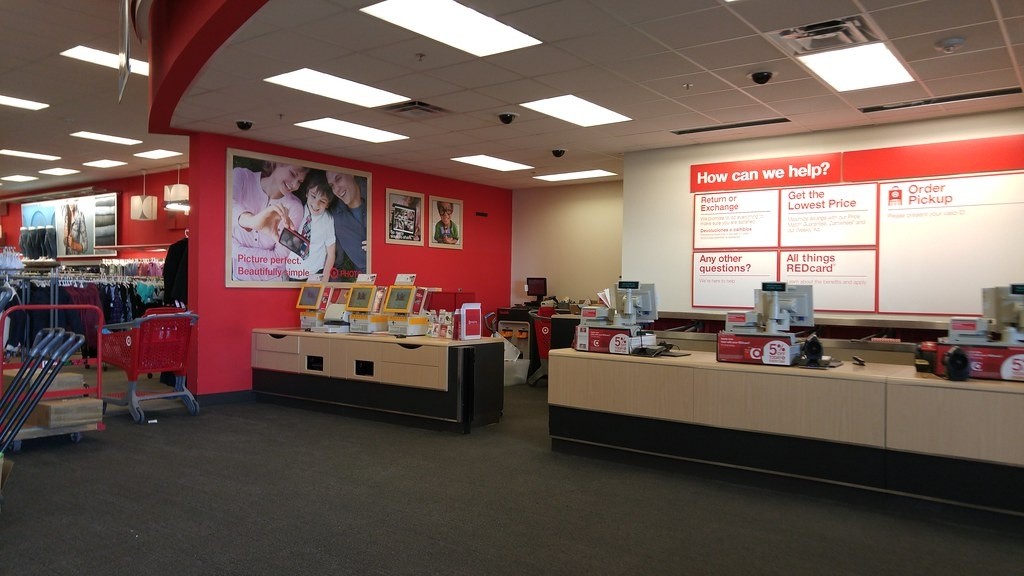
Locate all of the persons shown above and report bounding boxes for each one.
[63,200,88,255]
[27,226,37,261]
[391,196,420,241]
[45,225,56,261]
[37,226,46,261]
[326,171,367,281]
[232,162,311,281]
[435,201,458,244]
[19,227,28,261]
[286,174,336,281]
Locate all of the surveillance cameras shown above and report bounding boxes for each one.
[552,150,565,157]
[752,72,772,85]
[499,114,515,125]
[237,120,252,130]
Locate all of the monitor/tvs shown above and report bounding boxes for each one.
[758,285,815,327]
[995,285,1024,324]
[526,278,547,296]
[383,285,416,313]
[614,283,658,320]
[346,285,377,312]
[296,283,326,309]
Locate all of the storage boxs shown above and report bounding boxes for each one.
[504,358,530,387]
[2,368,83,426]
[28,398,104,428]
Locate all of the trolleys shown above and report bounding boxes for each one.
[0,304,106,450]
[82,307,201,424]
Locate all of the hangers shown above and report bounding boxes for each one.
[103,257,156,266]
[30,276,133,289]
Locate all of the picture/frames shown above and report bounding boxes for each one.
[429,195,463,249]
[225,147,372,288]
[385,188,424,246]
[22,193,118,260]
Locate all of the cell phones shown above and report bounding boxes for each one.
[279,227,310,259]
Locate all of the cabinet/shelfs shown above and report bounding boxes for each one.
[0,268,164,365]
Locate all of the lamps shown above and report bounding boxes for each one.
[130,170,157,221]
[163,165,189,211]
